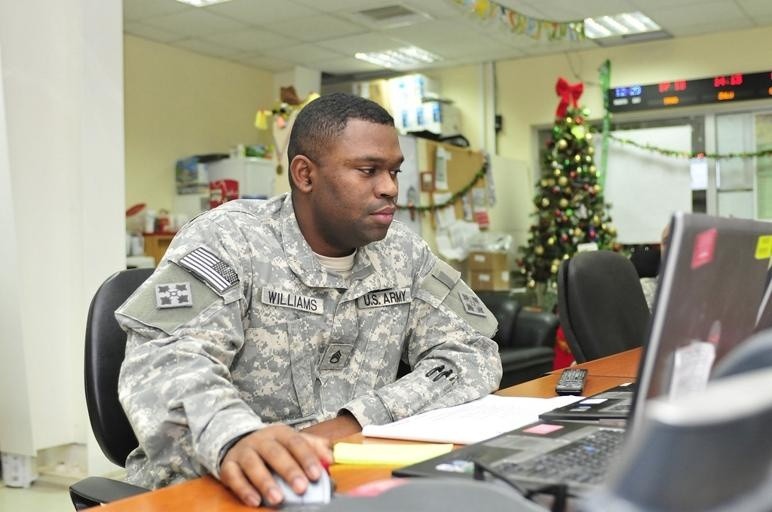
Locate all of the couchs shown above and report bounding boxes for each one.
[472,296,560,393]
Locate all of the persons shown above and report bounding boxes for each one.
[114,89,507,512]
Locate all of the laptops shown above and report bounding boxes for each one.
[538,277,772,421]
[390,210,772,512]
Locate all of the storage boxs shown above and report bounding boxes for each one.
[468,250,509,290]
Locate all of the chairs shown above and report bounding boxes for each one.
[84,268,157,468]
[557,247,651,365]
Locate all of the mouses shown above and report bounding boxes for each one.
[554,369,588,395]
[263,462,334,508]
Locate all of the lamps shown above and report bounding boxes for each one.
[568,11,675,50]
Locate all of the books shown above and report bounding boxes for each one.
[361,392,586,446]
